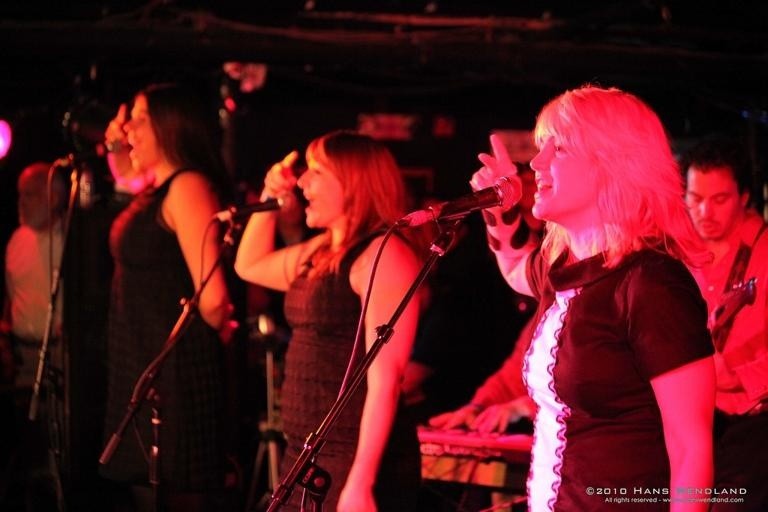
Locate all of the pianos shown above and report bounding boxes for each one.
[415,423,534,494]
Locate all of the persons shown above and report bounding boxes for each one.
[0,82,768,512]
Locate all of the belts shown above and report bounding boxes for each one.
[10,335,57,348]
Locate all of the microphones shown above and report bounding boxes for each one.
[60,138,120,172]
[396,176,524,229]
[209,193,296,222]
